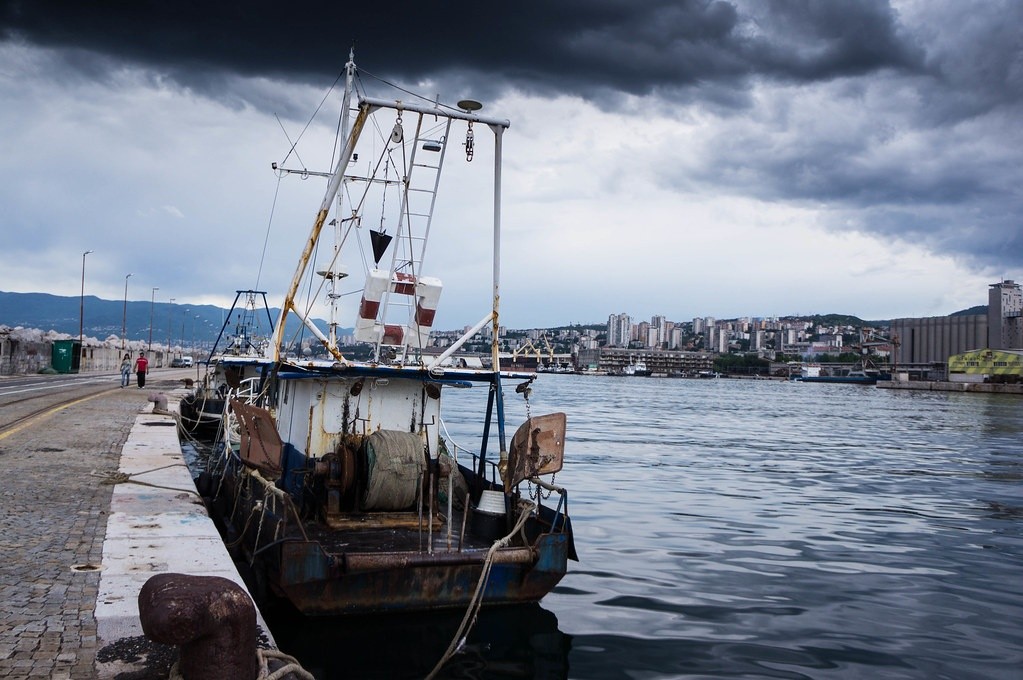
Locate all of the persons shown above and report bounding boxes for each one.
[120,353,132,389]
[134,352,149,389]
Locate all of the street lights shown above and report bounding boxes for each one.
[81,249,93,347]
[168,298,175,350]
[181,308,189,349]
[193,314,218,353]
[149,287,159,349]
[123,273,134,349]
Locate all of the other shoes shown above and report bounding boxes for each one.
[138,386,140,388]
[120,385,124,388]
[142,386,144,389]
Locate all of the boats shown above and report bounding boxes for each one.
[213,45,579,618]
[536,362,575,374]
[622,360,653,377]
[802,355,890,385]
[179,290,283,433]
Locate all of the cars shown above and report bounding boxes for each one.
[171,359,184,368]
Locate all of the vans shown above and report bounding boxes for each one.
[182,357,193,366]
